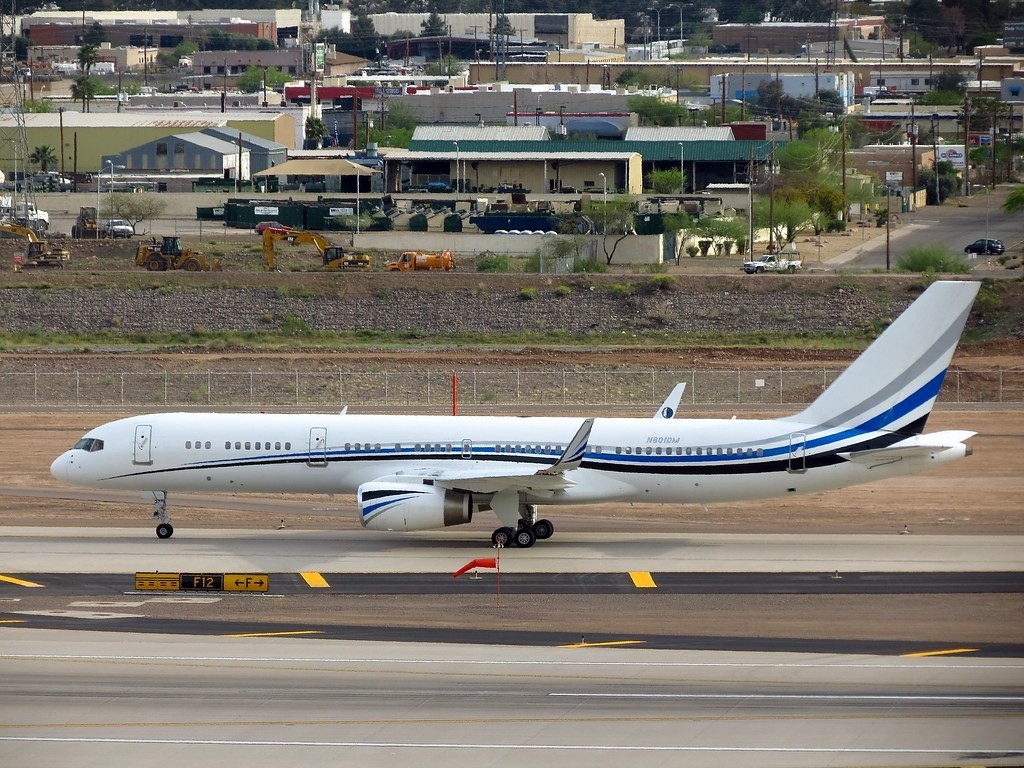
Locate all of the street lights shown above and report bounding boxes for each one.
[678,142,683,193]
[106,160,114,220]
[232,141,237,197]
[597,172,607,237]
[96,165,125,239]
[352,165,361,232]
[453,141,459,195]
[974,185,989,253]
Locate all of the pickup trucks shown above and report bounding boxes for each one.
[743,255,802,273]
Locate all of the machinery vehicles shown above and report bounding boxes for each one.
[134,235,223,272]
[0,220,71,269]
[72,206,106,239]
[262,226,371,272]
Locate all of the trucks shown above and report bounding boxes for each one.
[386,251,455,271]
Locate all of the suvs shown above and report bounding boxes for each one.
[965,238,1005,255]
[255,221,294,235]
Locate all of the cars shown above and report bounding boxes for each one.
[2,172,92,194]
[104,220,133,239]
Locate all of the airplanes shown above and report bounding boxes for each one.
[49,281,983,548]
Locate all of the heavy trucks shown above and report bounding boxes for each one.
[0,197,49,230]
